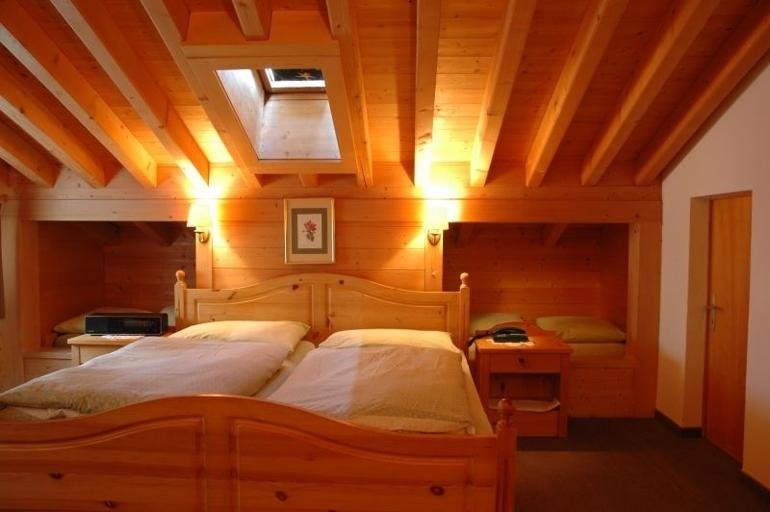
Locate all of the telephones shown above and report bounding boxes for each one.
[493,327,529,342]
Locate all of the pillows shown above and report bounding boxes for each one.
[535,312,626,343]
[167,319,463,356]
[51,304,155,349]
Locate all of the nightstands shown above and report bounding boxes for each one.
[471,334,574,441]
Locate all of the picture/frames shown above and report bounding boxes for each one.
[283,196,337,266]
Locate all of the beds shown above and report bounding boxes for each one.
[1,266,519,512]
